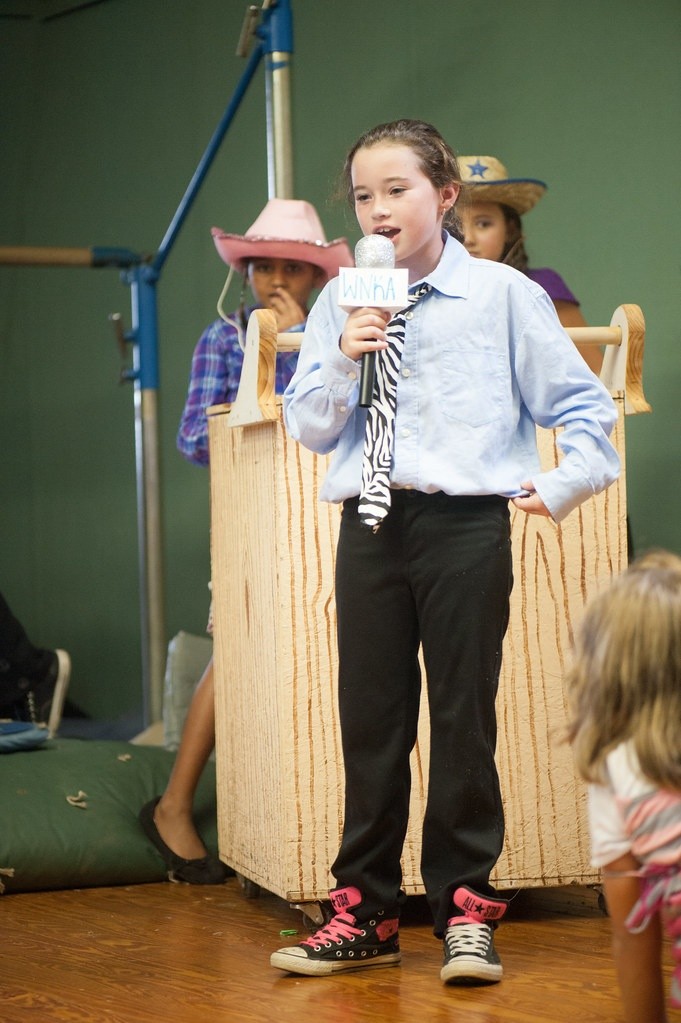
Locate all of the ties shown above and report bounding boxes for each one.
[356,282,434,535]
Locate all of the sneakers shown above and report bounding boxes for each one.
[270,883,401,977]
[440,884,509,987]
[22,648,74,739]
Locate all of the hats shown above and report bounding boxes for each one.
[211,197,356,282]
[456,155,547,217]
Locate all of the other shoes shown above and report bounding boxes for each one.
[138,795,224,884]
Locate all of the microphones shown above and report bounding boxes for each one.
[337,234,410,407]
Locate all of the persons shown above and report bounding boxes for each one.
[269,120,622,988]
[445,155,605,380]
[136,200,352,885]
[0,593,72,739]
[543,554,681,1023]
